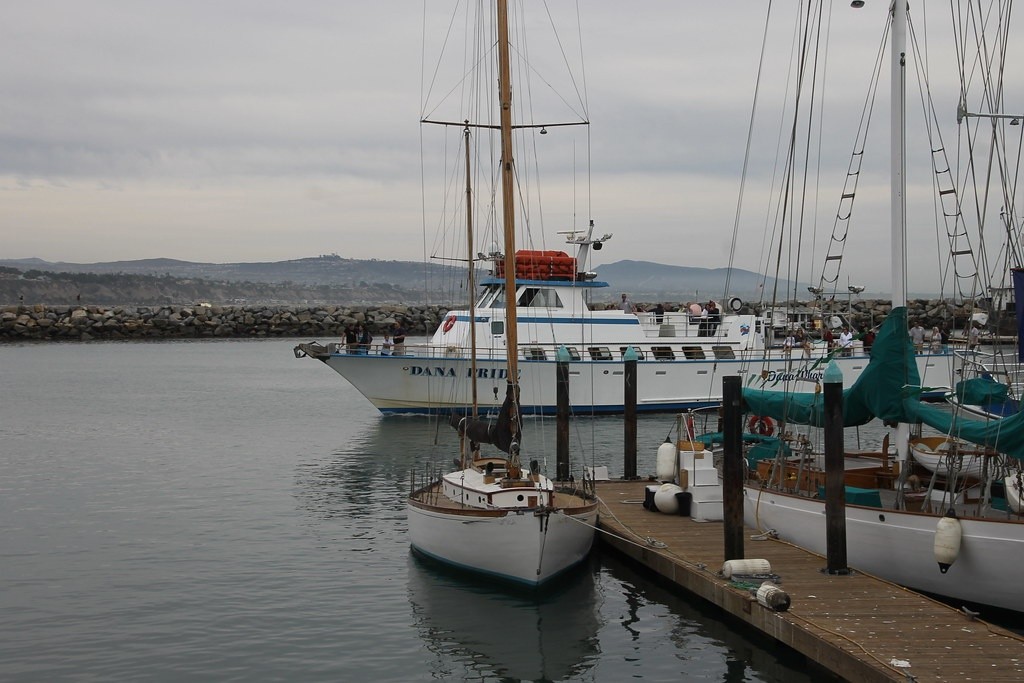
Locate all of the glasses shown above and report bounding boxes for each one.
[844,329,846,330]
[396,324,397,325]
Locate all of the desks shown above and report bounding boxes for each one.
[693,317,713,337]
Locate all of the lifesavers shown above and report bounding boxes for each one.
[443,316,456,332]
[749,417,774,437]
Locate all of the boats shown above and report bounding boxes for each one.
[294,221,875,418]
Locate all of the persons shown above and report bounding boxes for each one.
[393,320,405,355]
[823,325,880,356]
[616,294,721,337]
[782,327,816,355]
[381,335,394,356]
[932,322,949,354]
[969,324,979,351]
[341,323,373,355]
[910,320,926,355]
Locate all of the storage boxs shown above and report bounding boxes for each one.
[680,440,704,451]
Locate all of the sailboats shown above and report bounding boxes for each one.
[405,0,600,597]
[670,0,1024,634]
